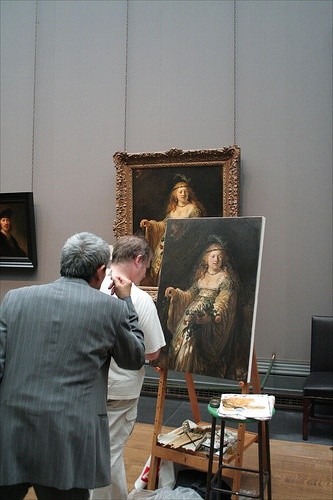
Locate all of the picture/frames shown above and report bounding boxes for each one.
[143,216,266,382]
[0,192,37,271]
[113,144,242,302]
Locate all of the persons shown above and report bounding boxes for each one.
[86,236,166,500]
[0,232,146,500]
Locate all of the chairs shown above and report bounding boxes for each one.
[302,314,333,441]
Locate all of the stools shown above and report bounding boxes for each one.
[205,398,276,500]
